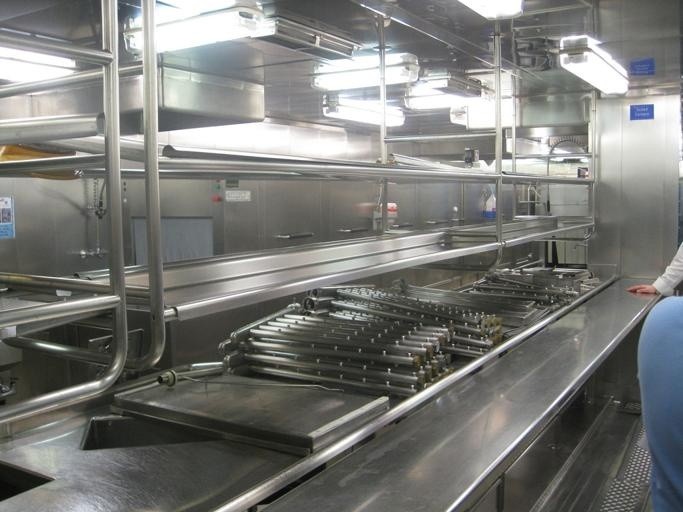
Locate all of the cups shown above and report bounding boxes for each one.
[387,201,398,219]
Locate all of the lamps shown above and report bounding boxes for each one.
[558,34,631,80]
[315,50,423,74]
[311,61,484,128]
[560,49,630,101]
[1,45,80,83]
[122,1,280,59]
[450,94,521,130]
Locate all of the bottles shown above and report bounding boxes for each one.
[486,193,496,212]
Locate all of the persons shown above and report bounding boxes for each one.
[625,241,683,296]
